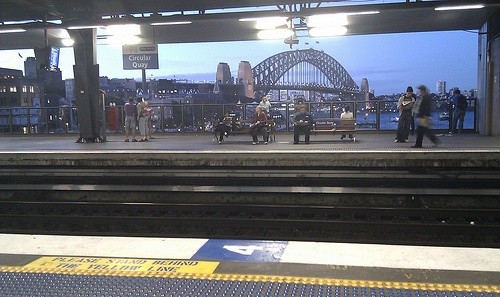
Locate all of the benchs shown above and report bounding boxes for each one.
[213,120,275,143]
[310,118,356,143]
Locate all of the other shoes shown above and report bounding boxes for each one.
[405,139,409,143]
[132,138,139,142]
[411,144,422,148]
[393,138,398,142]
[416,117,429,128]
[139,138,146,142]
[449,129,457,133]
[252,142,257,145]
[264,142,268,144]
[125,139,129,142]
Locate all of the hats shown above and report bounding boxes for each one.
[406,86,413,92]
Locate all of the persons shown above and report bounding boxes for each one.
[258,96,272,114]
[213,120,228,144]
[136,96,155,142]
[293,105,313,144]
[340,106,354,139]
[393,85,442,148]
[124,97,138,142]
[251,106,269,145]
[450,89,468,135]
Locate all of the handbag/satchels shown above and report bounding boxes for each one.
[137,103,150,116]
